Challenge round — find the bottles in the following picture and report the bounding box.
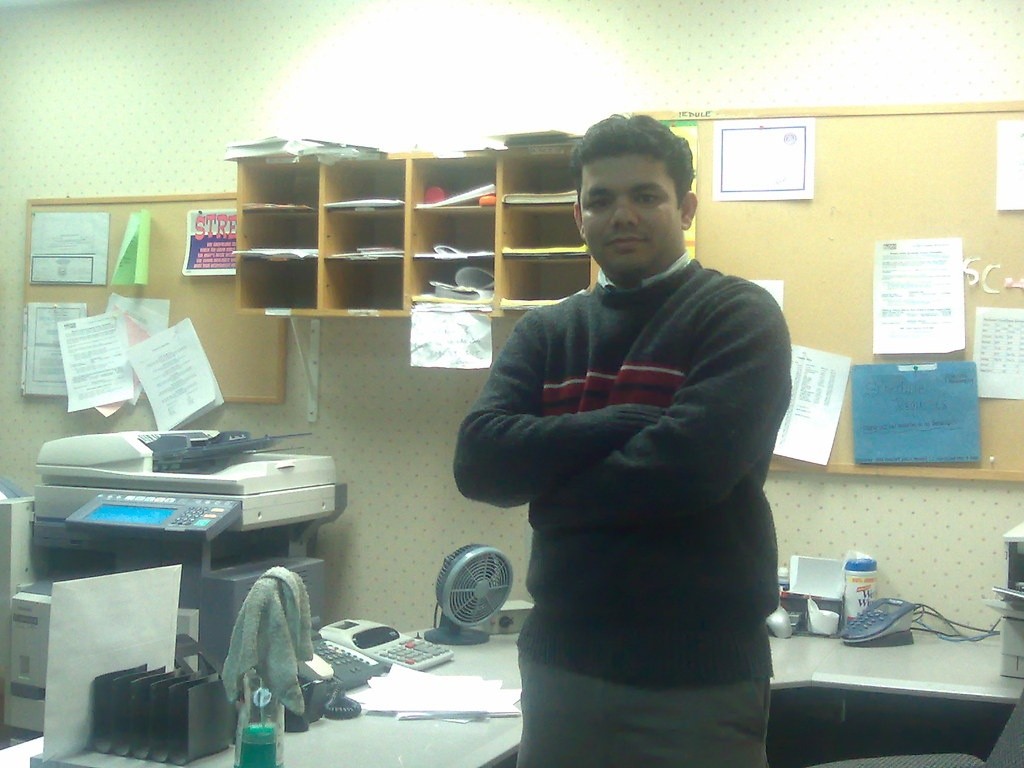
[777,568,789,591]
[235,673,285,768]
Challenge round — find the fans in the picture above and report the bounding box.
[424,545,513,645]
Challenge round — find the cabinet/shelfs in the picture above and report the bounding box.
[234,152,603,318]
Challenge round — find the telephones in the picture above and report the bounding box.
[297,629,373,694]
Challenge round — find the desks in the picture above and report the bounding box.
[0,625,1024,768]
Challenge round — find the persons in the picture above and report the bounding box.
[453,112,792,768]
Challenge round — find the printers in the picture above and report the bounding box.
[8,429,348,747]
[992,522,1024,679]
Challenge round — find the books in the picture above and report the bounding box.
[226,130,587,313]
